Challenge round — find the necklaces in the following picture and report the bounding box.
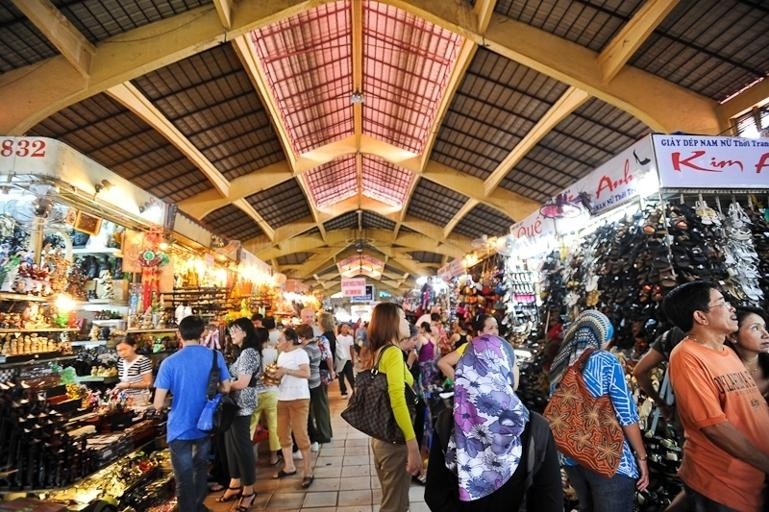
[739,354,762,372]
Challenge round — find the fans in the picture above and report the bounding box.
[471,232,495,254]
[493,232,522,256]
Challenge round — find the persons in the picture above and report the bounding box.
[659,279,769,512]
[722,305,768,404]
[631,324,690,512]
[108,337,152,403]
[154,302,647,512]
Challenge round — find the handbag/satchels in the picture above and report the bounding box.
[541,346,626,481]
[195,348,239,438]
[340,342,420,447]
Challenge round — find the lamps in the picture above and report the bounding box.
[92,178,113,201]
[209,235,228,249]
[137,201,158,218]
[538,187,595,220]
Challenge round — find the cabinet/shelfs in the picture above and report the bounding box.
[0,293,81,371]
[65,245,128,348]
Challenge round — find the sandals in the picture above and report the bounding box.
[215,485,242,503]
[235,490,258,512]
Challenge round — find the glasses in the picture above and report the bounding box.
[708,300,732,310]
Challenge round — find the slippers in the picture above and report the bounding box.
[268,454,315,488]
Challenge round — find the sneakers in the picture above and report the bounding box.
[292,450,303,460]
[310,442,319,452]
